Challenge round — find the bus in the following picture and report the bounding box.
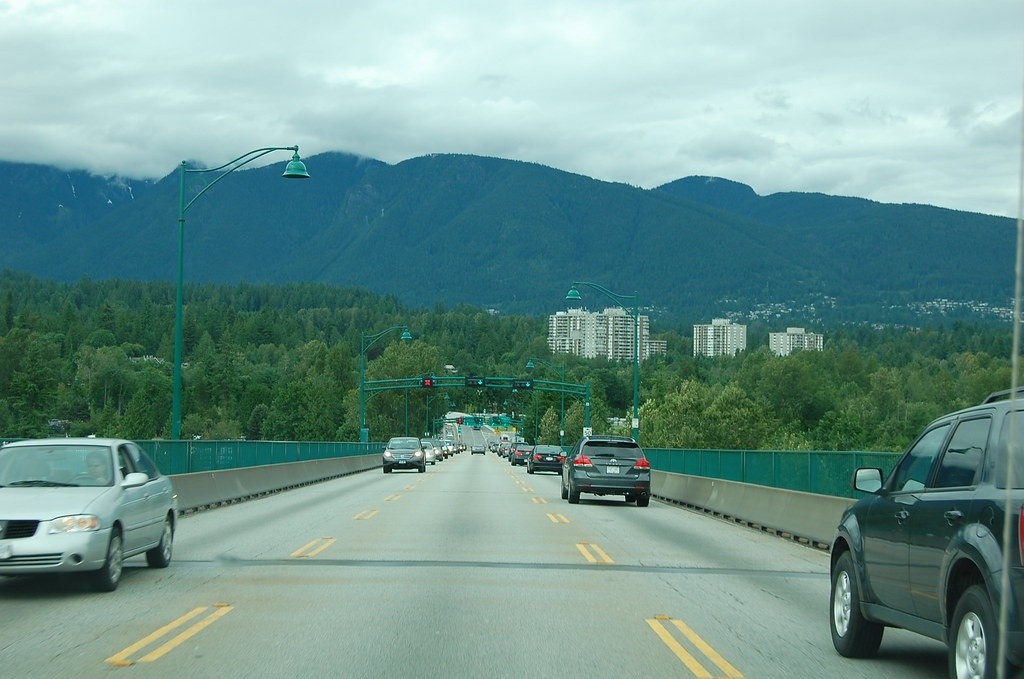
[560,435,652,507]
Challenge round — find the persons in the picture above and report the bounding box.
[72,451,113,486]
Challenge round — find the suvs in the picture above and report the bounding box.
[382,437,427,474]
[509,442,529,462]
[828,385,1024,679]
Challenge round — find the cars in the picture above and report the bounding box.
[488,441,509,456]
[526,444,565,474]
[439,440,449,459]
[426,437,443,461]
[504,446,511,458]
[511,445,535,467]
[0,437,179,591]
[471,445,486,455]
[420,441,437,465]
[443,425,467,455]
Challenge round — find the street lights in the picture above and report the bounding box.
[359,326,413,442]
[172,144,311,443]
[566,281,640,442]
[526,356,564,446]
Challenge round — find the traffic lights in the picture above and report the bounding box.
[458,418,462,424]
[513,378,534,388]
[422,377,434,387]
[465,376,485,387]
